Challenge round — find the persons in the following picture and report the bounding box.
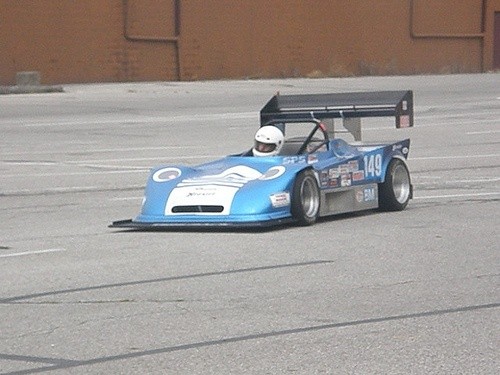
[252,124,285,158]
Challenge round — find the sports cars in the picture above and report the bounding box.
[107,90,415,231]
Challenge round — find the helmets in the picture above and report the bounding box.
[251,125,284,157]
